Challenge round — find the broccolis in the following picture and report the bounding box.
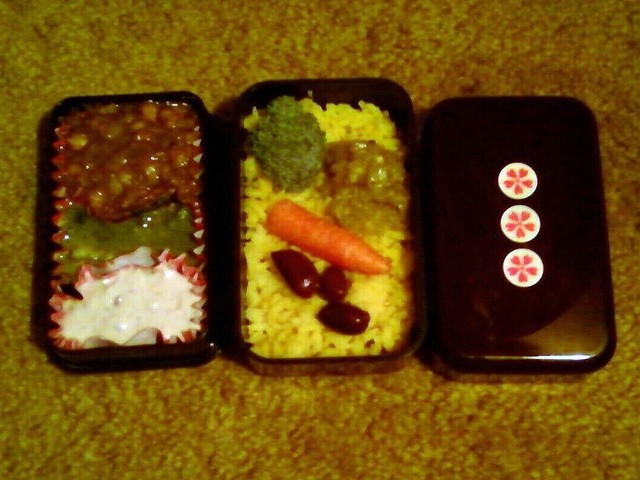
[254,97,325,193]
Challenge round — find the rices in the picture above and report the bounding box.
[239,95,414,359]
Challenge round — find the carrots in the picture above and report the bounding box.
[264,200,392,276]
